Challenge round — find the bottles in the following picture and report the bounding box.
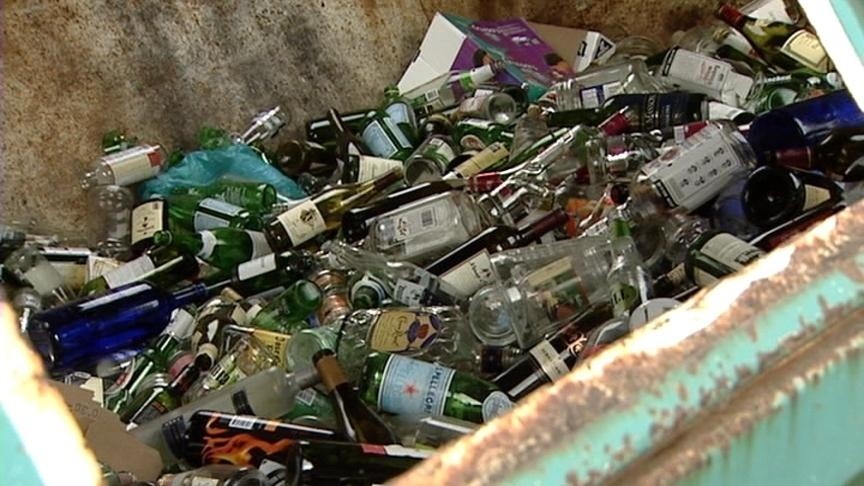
[2,1,863,485]
